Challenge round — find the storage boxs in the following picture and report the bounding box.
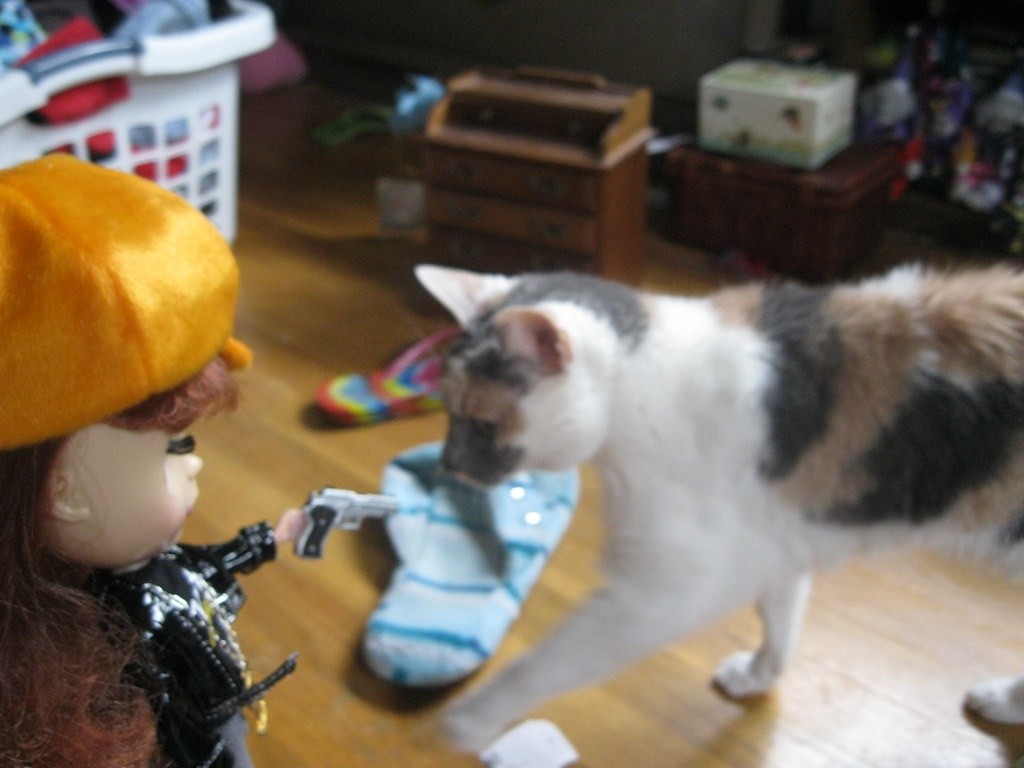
[668,136,904,289]
[700,59,859,162]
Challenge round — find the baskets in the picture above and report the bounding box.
[0,0,278,245]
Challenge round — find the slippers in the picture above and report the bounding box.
[361,441,576,686]
[315,326,471,426]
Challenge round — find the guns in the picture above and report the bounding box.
[293,485,401,559]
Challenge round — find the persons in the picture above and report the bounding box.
[0,151,304,768]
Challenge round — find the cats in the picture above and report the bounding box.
[406,259,1024,729]
